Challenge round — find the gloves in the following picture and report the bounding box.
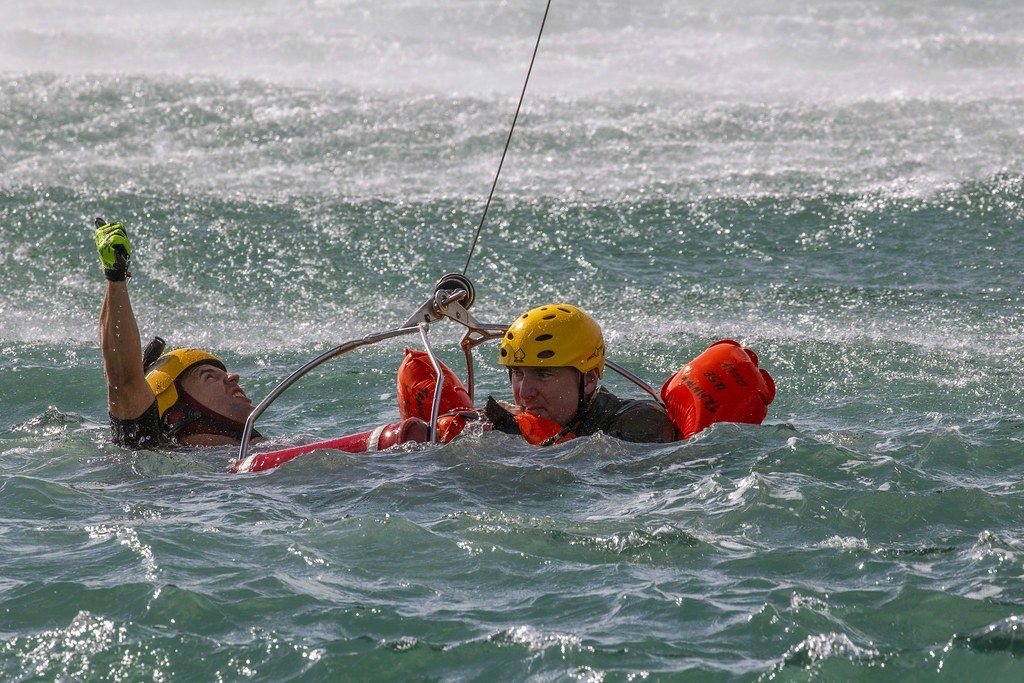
[95,217,131,281]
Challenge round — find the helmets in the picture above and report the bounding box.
[497,303,605,380]
[143,336,227,420]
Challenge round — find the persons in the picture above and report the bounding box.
[488,302,674,448]
[93,215,273,450]
